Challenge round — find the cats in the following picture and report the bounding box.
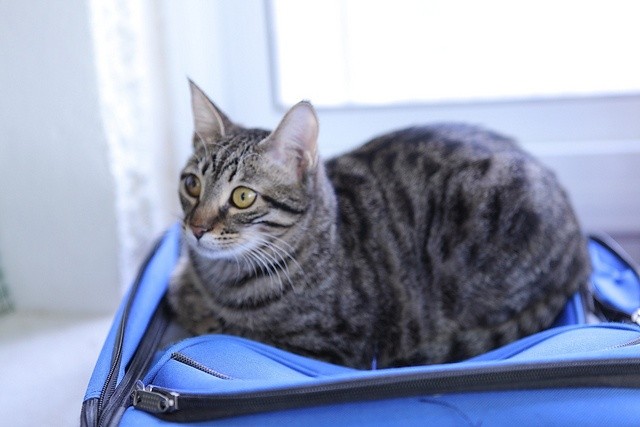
[166,76,594,372]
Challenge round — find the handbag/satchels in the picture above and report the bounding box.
[80,224,640,427]
